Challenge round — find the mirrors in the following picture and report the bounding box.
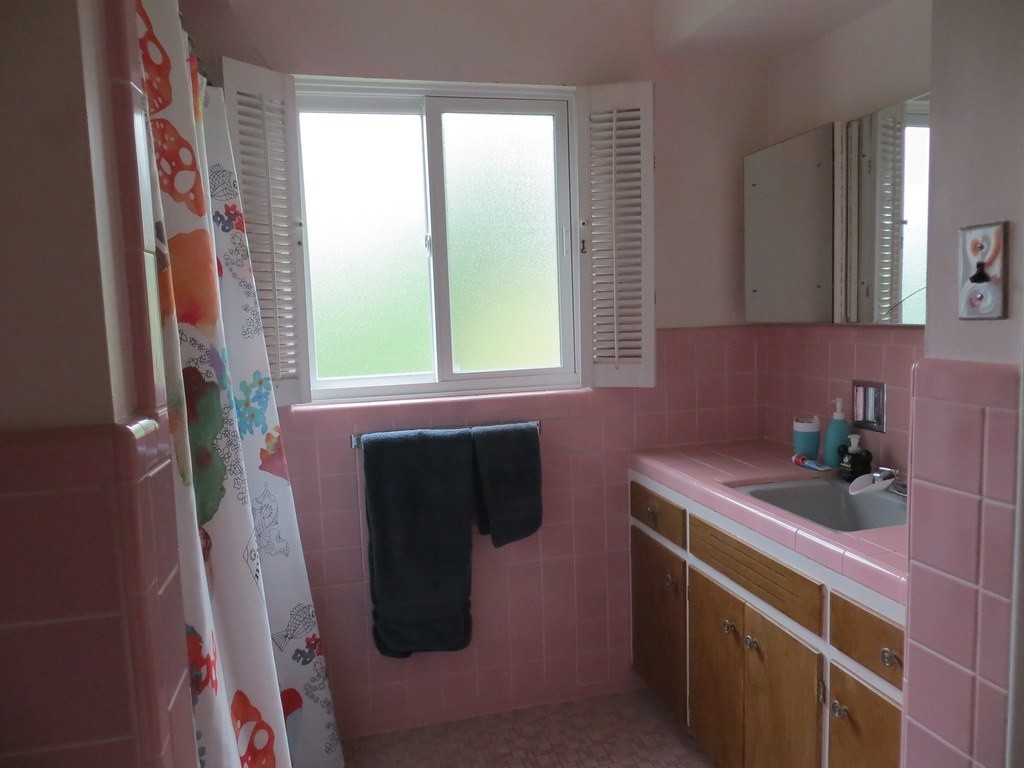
[845,91,931,326]
[743,122,835,324]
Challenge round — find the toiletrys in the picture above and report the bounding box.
[791,395,873,483]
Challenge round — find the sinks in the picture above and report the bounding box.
[730,475,908,534]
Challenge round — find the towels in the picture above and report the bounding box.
[360,421,544,660]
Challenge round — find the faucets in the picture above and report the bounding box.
[872,468,907,496]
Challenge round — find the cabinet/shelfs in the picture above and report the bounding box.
[686,510,826,768]
[627,468,687,729]
[826,569,907,768]
